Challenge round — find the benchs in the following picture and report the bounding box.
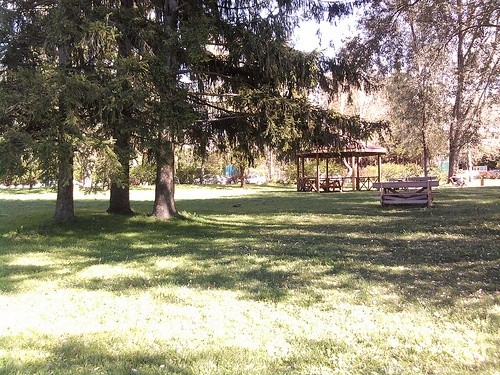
[318,179,343,193]
[372,181,440,211]
[388,175,438,203]
